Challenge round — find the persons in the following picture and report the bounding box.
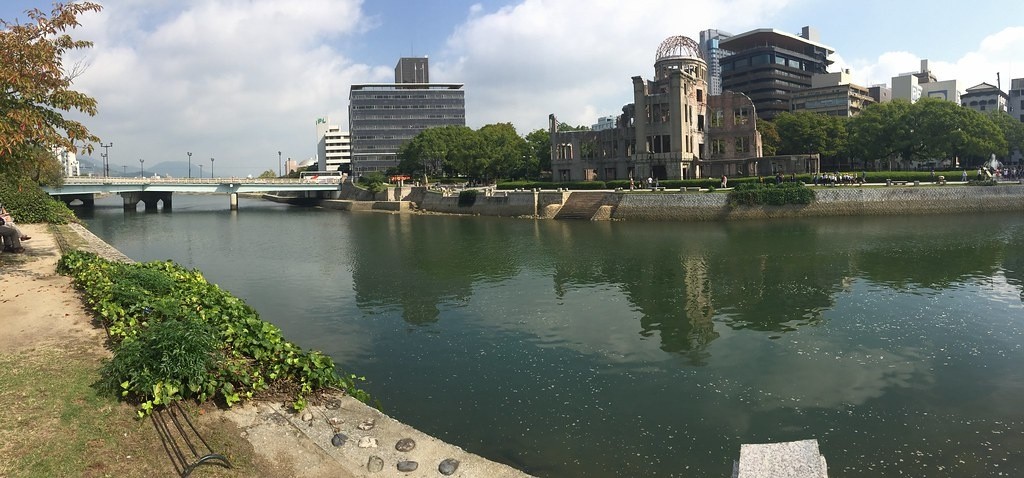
[630,176,659,190]
[721,175,727,188]
[0,207,32,254]
[435,179,476,187]
[759,171,866,187]
[931,164,1024,183]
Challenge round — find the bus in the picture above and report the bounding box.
[300,171,343,183]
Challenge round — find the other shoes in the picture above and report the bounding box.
[20,235,31,241]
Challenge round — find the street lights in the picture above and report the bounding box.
[100,142,113,177]
[187,152,193,178]
[140,158,145,178]
[211,158,214,178]
[278,150,282,177]
[101,152,107,177]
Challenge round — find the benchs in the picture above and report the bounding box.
[892,181,908,185]
[493,191,506,196]
[687,187,701,191]
[648,187,665,191]
